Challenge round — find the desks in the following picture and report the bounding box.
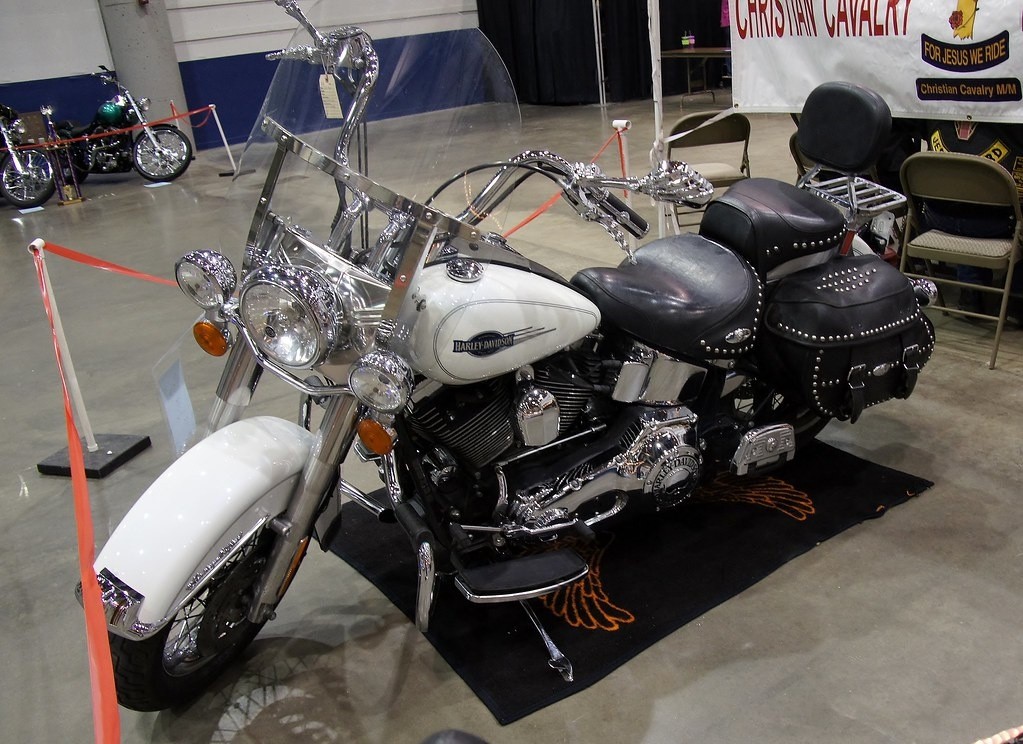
[661,48,732,110]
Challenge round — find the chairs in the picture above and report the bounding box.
[789,129,881,188]
[661,112,752,230]
[895,151,1023,370]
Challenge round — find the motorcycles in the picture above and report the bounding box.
[74,0,935,717]
[48,65,194,186]
[0,105,57,210]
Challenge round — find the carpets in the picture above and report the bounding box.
[326,435,937,726]
